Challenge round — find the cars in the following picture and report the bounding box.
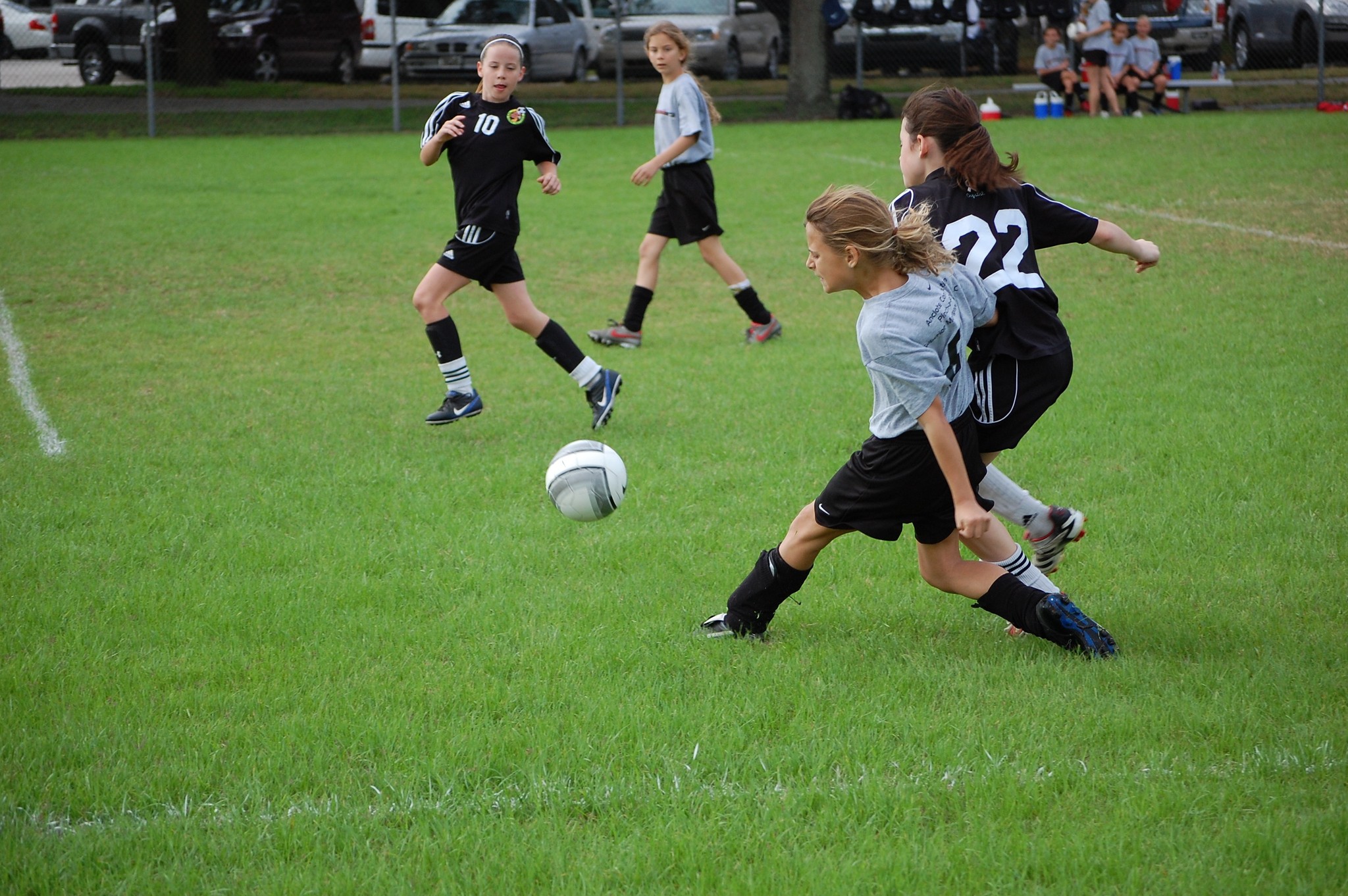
[1221,1,1348,69]
[401,0,593,84]
[565,0,627,66]
[1023,1,1230,70]
[50,0,170,85]
[138,0,365,88]
[834,0,1021,77]
[594,0,784,83]
[357,0,457,76]
[0,0,54,60]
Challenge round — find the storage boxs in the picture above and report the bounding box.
[1168,55,1182,79]
[1034,90,1065,118]
[981,97,1001,120]
[1164,90,1181,112]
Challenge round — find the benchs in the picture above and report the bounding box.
[1012,78,1233,112]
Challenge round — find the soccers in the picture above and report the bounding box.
[543,439,628,523]
[516,105,527,117]
[1064,20,1089,42]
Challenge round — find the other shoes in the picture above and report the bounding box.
[1131,109,1143,117]
[1081,100,1090,111]
[1101,110,1109,117]
[1064,109,1074,115]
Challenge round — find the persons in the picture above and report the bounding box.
[413,36,622,429]
[588,19,785,350]
[699,185,1121,663]
[888,87,1160,637]
[1034,0,1167,116]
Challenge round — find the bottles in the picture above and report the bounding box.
[1218,61,1227,80]
[1211,61,1217,79]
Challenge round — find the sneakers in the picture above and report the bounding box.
[746,312,782,341]
[1002,621,1025,637]
[1024,505,1089,577]
[700,613,763,639]
[588,318,641,348]
[424,388,482,425]
[1036,593,1120,658]
[584,368,623,429]
